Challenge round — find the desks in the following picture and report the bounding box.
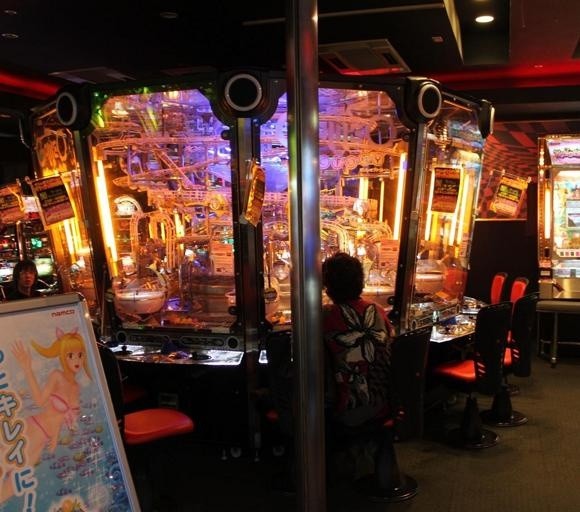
[536,300,580,367]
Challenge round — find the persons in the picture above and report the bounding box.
[322,252,395,491]
[0,262,45,302]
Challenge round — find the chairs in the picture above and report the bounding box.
[100,346,197,512]
